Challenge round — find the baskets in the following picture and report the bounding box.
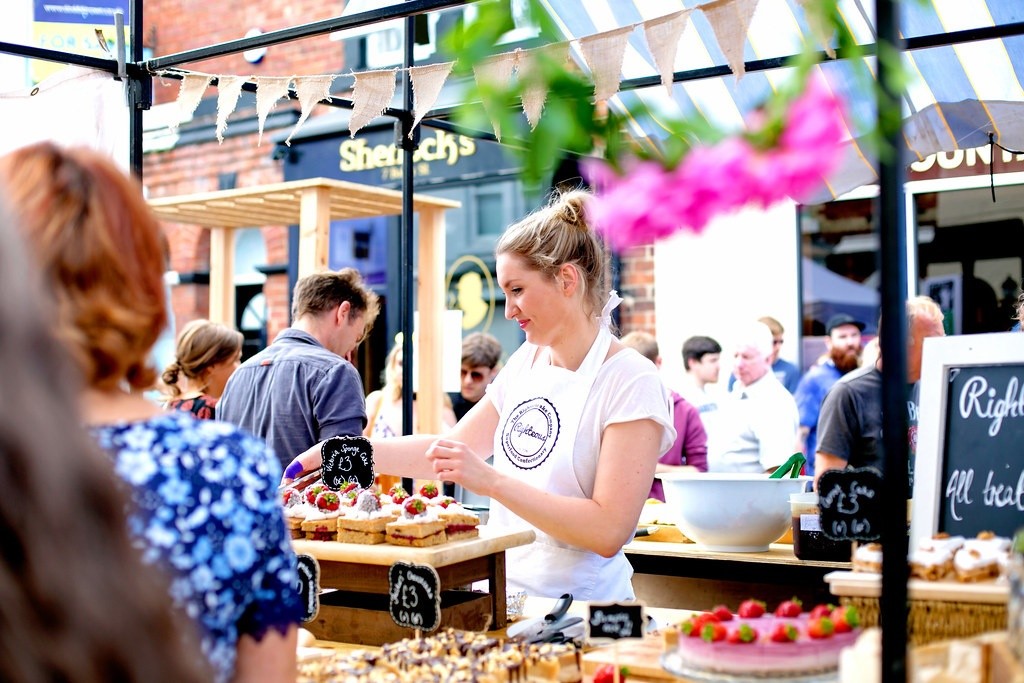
[836,594,1008,655]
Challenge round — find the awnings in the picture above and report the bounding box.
[541,0,1024,205]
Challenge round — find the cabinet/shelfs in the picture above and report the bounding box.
[291,527,535,649]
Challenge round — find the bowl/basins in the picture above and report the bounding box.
[655,472,808,552]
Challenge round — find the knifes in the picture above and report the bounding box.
[506,593,573,643]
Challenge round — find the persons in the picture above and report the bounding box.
[281,188,676,601]
[0,138,304,683]
[816,299,946,496]
[362,333,502,437]
[216,270,379,472]
[162,320,243,420]
[0,191,210,683]
[621,315,869,505]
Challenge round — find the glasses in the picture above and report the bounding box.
[461,368,491,383]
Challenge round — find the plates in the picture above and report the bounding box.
[507,616,586,644]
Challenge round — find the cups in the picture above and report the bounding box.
[789,493,851,561]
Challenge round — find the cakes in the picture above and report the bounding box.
[666,600,865,683]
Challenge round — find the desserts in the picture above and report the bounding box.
[852,542,882,574]
[280,483,480,548]
[908,532,1012,583]
[296,627,582,683]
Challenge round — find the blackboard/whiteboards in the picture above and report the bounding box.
[909,331,1024,554]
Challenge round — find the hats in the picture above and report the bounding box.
[825,313,866,336]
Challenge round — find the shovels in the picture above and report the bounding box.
[510,592,573,643]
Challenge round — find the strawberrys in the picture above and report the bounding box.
[593,665,626,683]
